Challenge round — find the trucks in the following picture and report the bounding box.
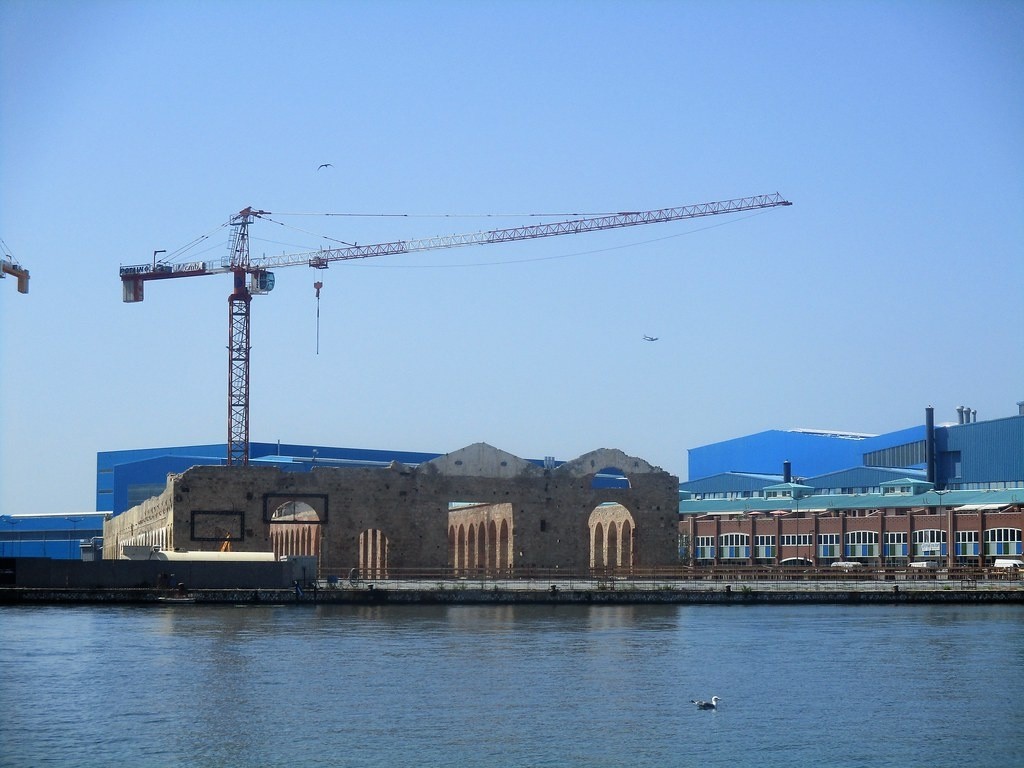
[906,562,940,568]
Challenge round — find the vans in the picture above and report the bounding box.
[994,559,1024,567]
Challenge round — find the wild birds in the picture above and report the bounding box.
[690,696,722,710]
[317,163,336,171]
[642,333,661,342]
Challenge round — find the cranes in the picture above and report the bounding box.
[118,189,793,469]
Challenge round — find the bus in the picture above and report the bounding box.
[831,561,862,568]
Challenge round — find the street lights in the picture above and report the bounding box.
[786,492,811,580]
[929,488,952,578]
